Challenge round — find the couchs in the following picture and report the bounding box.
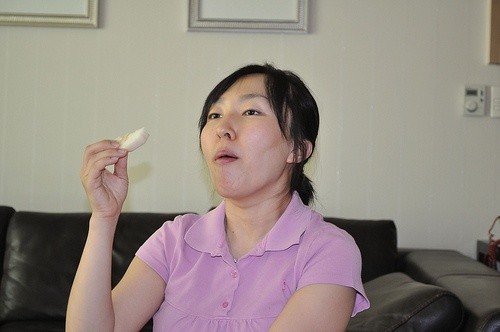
[0,206,500,332]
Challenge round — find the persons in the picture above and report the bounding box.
[64,63,371,332]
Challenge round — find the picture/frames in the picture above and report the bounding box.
[0,0,98,30]
[183,0,310,35]
